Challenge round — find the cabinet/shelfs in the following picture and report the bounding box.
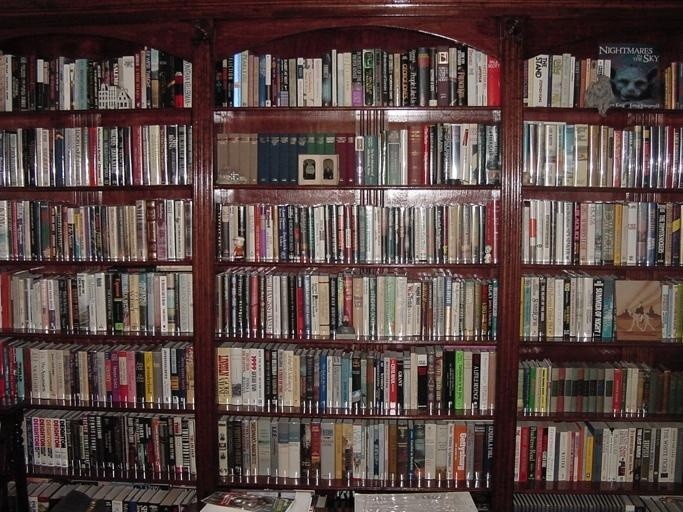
[0,1,202,496]
[513,0,683,496]
[200,2,511,497]
